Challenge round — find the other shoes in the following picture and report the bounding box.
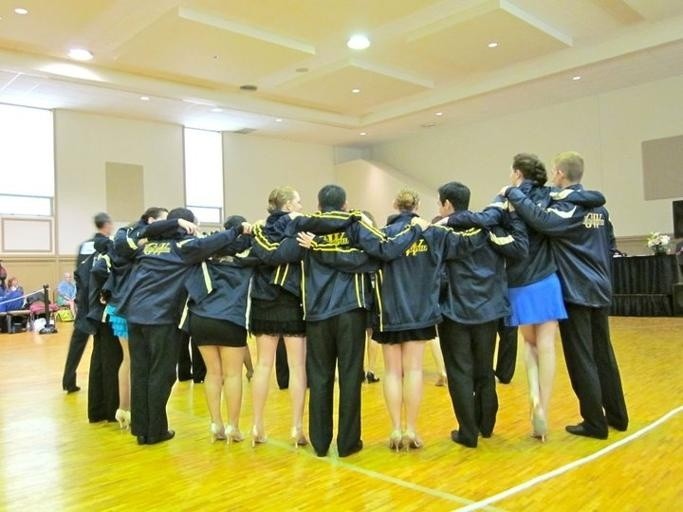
[89,415,116,423]
[314,447,329,456]
[340,440,362,456]
[138,430,175,444]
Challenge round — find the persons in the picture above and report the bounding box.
[250,152,630,458]
[62,187,362,450]
[1,266,24,312]
[57,272,77,319]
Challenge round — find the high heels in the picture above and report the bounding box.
[250,425,266,447]
[434,372,447,387]
[367,371,380,383]
[291,429,307,448]
[209,422,227,443]
[389,430,403,452]
[402,431,420,453]
[115,410,131,430]
[224,424,245,445]
[532,417,548,442]
[245,371,252,383]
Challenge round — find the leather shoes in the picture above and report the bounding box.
[63,383,80,392]
[604,416,627,431]
[566,422,607,440]
[452,430,477,448]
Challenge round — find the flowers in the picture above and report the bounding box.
[639,231,672,248]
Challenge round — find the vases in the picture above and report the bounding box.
[653,246,669,255]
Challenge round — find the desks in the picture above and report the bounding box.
[608,253,682,318]
